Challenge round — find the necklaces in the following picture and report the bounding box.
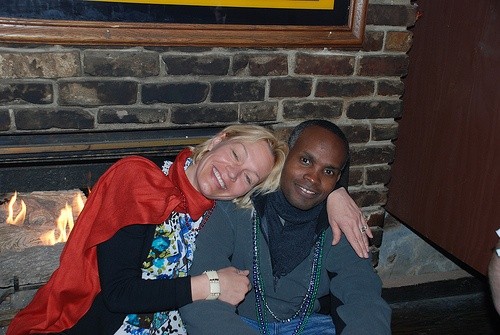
[173,187,217,232]
[252,209,326,335]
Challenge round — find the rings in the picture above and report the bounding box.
[360,225,367,233]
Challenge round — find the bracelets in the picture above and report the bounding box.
[202,270,221,301]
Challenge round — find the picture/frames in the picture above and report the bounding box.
[0,0,368,49]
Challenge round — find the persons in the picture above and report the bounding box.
[6,123,374,335]
[178,120,391,335]
